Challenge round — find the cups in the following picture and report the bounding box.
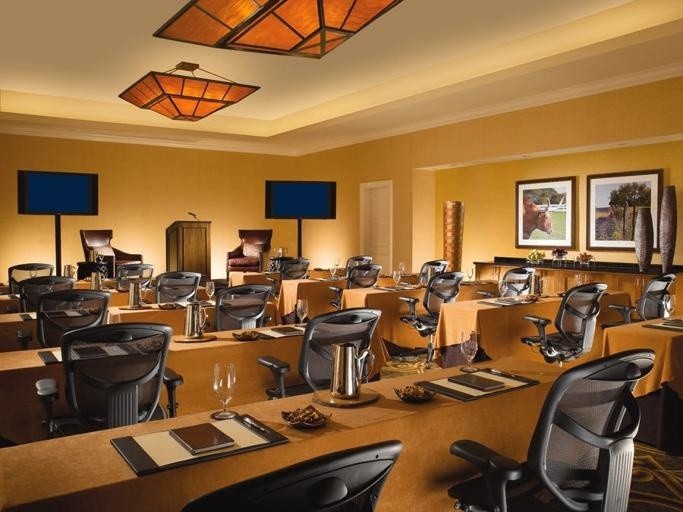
[278,247,289,257]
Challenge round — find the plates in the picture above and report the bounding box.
[232,331,265,341]
[279,404,333,429]
[117,289,129,293]
[158,304,176,309]
[394,383,437,403]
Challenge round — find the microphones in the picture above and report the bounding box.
[188,212,201,222]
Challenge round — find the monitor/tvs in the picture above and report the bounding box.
[16,168,99,216]
[263,178,337,219]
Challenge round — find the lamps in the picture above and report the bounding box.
[117,70,261,123]
[150,1,400,61]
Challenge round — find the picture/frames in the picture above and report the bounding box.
[514,175,575,249]
[586,168,663,252]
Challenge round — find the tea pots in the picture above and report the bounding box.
[63,264,76,277]
[183,302,209,339]
[330,341,375,399]
[127,280,147,308]
[527,273,547,299]
[89,271,106,291]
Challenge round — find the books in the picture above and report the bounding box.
[271,326,303,336]
[46,311,68,318]
[448,373,504,392]
[169,423,235,455]
[73,345,107,359]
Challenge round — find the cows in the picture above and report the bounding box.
[523,196,553,239]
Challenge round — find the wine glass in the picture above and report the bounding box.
[106,311,121,325]
[330,255,341,278]
[296,299,309,326]
[460,330,478,372]
[393,263,405,287]
[662,294,678,322]
[211,362,237,419]
[466,267,474,282]
[498,280,508,300]
[206,281,215,302]
[30,267,38,279]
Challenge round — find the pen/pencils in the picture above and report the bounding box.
[490,370,516,379]
[243,417,270,433]
